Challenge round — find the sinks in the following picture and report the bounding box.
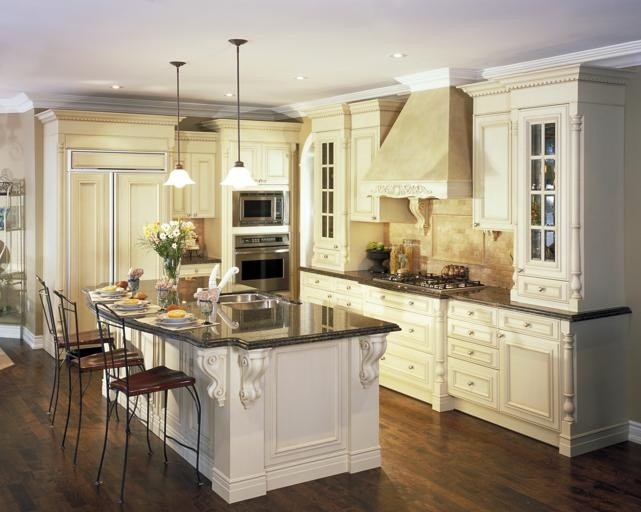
[217,291,269,304]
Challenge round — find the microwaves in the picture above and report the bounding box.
[232,190,290,226]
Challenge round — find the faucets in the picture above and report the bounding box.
[208,262,240,302]
[209,303,240,336]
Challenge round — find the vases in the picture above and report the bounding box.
[158,255,186,314]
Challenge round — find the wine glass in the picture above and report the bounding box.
[199,300,214,325]
[157,289,169,313]
[366,249,390,273]
[127,278,140,299]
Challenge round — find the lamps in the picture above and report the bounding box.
[163,60,197,188]
[219,36,258,190]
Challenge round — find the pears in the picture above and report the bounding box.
[135,291,147,300]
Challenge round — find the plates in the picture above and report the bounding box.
[114,300,144,307]
[97,288,124,295]
[94,292,125,297]
[161,313,191,322]
[109,304,150,310]
[153,319,198,325]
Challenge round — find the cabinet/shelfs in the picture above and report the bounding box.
[493,307,631,459]
[361,276,488,418]
[171,128,216,220]
[296,264,394,319]
[346,98,416,224]
[457,72,517,237]
[195,119,304,194]
[443,296,496,419]
[38,113,176,364]
[308,104,385,274]
[507,64,630,318]
[0,179,26,346]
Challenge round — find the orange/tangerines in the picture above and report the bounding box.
[366,242,390,252]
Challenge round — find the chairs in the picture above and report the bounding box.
[51,287,152,465]
[92,300,208,509]
[30,271,120,428]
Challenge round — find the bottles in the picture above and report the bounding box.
[389,238,418,272]
[443,263,470,278]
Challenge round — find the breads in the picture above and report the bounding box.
[167,309,186,319]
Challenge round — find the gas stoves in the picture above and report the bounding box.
[373,269,486,294]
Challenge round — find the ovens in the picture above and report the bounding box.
[232,234,290,292]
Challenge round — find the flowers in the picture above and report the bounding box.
[134,216,205,285]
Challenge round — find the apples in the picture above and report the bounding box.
[119,281,128,288]
